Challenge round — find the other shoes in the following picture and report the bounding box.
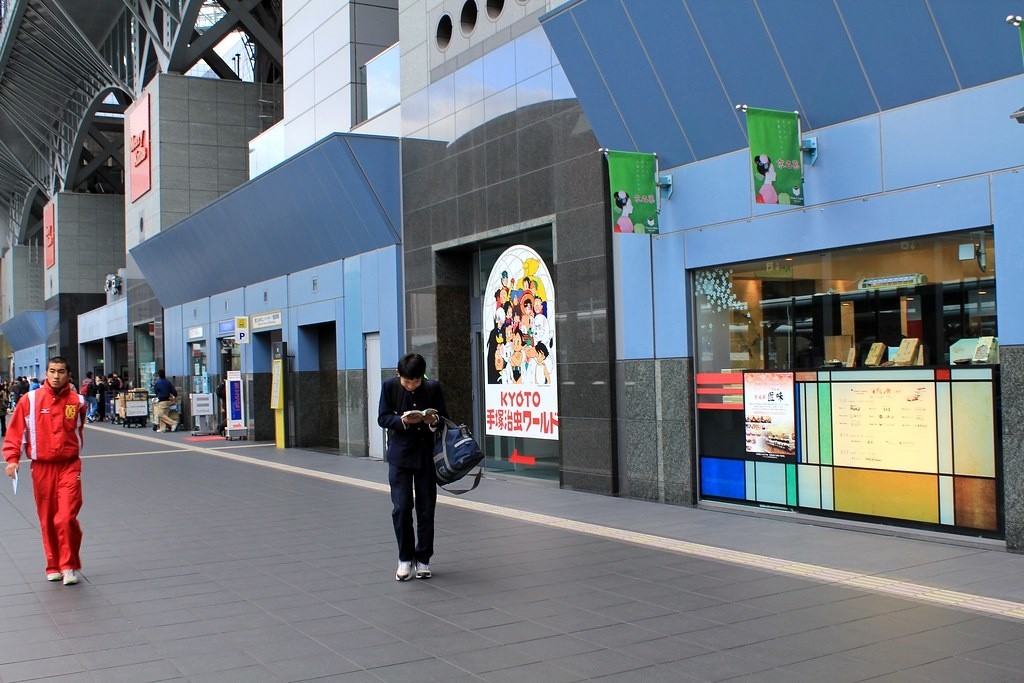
[96,418,103,421]
[156,429,166,433]
[87,415,94,423]
[171,421,178,432]
[110,418,120,424]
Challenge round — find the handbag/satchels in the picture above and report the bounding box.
[431,423,486,487]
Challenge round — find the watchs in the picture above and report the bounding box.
[431,414,438,426]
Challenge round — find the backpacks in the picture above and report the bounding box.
[78,381,91,398]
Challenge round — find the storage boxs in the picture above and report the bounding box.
[894,338,921,365]
[846,348,856,367]
[972,336,997,363]
[865,342,886,367]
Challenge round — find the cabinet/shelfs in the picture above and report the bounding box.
[113,389,148,427]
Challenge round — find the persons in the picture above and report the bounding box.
[1,356,87,586]
[67,371,124,424]
[377,353,446,582]
[0,376,45,437]
[153,369,179,433]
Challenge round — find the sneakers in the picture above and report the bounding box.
[395,557,416,581]
[62,569,79,585]
[47,572,61,581]
[414,561,431,578]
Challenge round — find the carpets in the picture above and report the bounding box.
[180,435,226,441]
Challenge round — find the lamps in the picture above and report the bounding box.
[858,273,927,290]
